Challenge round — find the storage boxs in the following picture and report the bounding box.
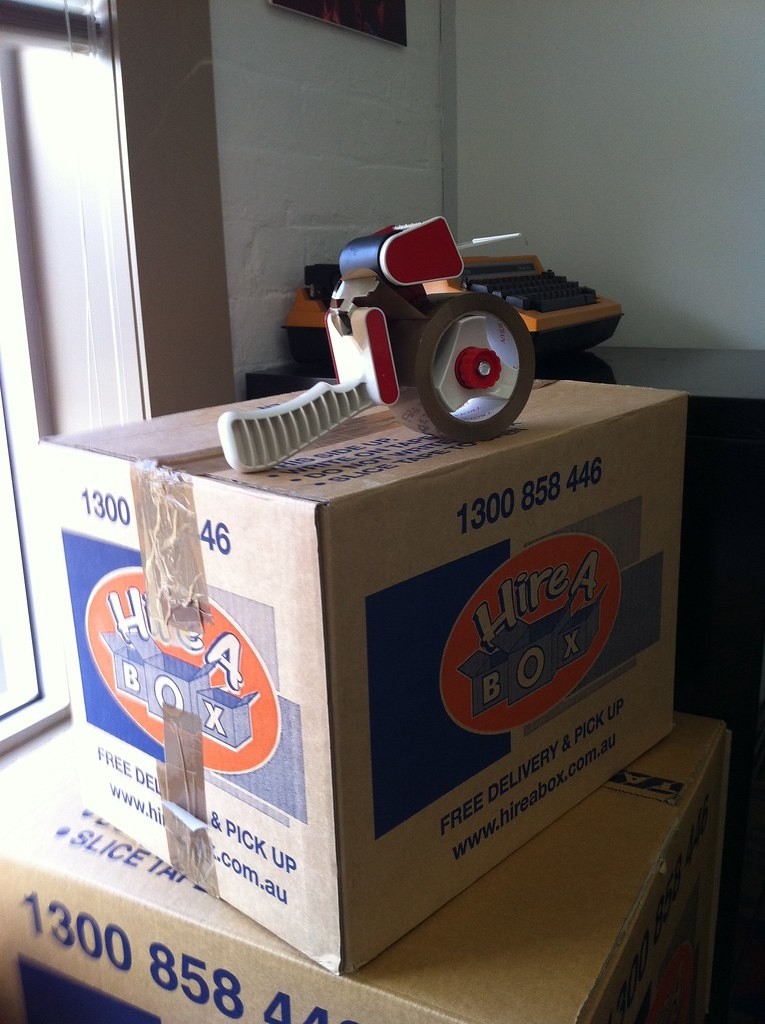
[2,682,738,1024]
[35,361,698,985]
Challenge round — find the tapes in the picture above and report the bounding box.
[387,292,535,444]
